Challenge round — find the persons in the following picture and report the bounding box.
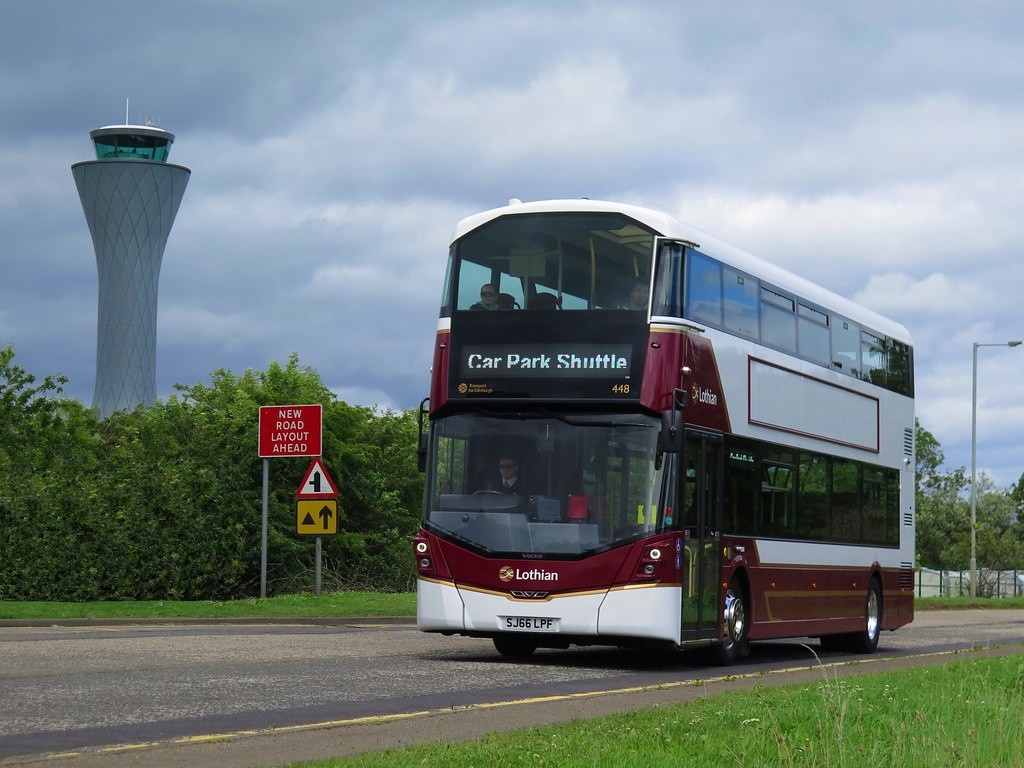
[616,276,650,310]
[483,450,529,514]
[469,284,507,311]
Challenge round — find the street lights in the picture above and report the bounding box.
[967,339,1022,599]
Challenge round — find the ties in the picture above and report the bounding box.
[504,482,509,488]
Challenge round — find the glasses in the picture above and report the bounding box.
[480,290,498,297]
[500,465,516,470]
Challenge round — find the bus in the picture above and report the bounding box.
[413,199,917,666]
